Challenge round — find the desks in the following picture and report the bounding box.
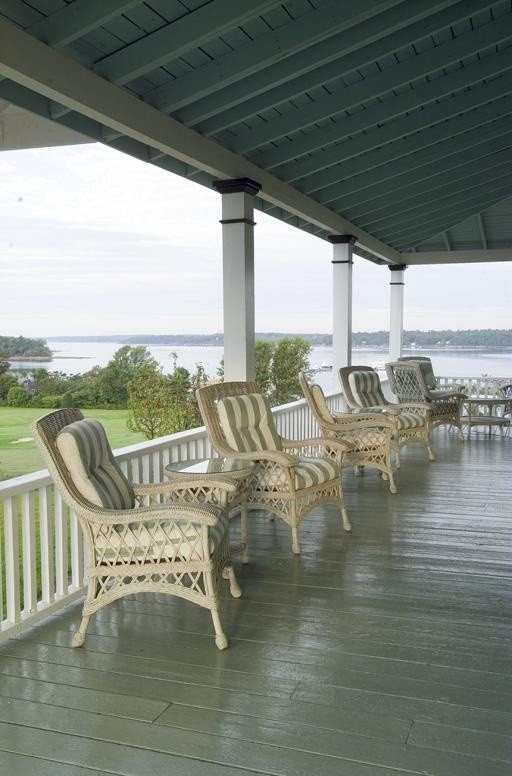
[161,454,255,564]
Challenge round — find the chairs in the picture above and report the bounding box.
[498,380,512,427]
[385,355,465,439]
[297,371,401,494]
[36,405,244,650]
[195,380,352,554]
[338,364,435,469]
